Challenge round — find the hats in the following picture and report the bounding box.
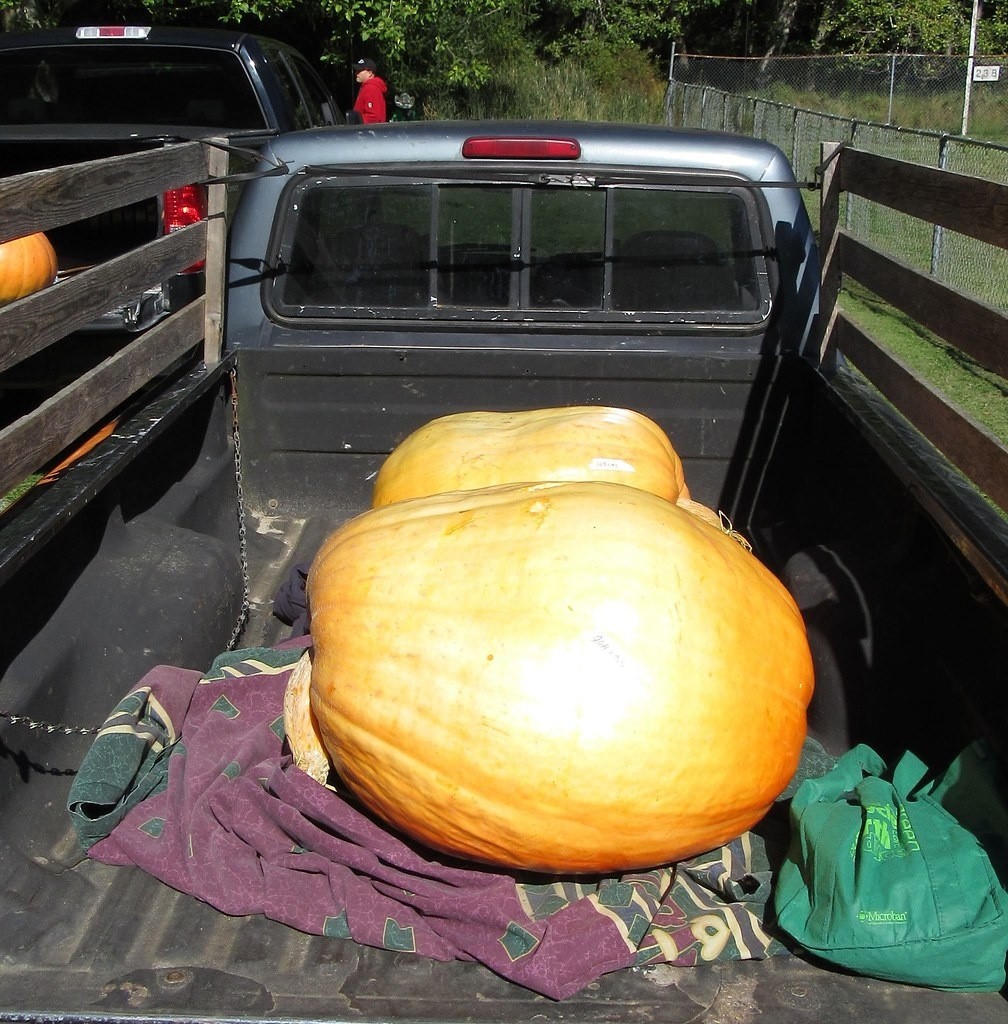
[352,59,377,72]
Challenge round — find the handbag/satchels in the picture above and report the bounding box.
[773,740,1008,992]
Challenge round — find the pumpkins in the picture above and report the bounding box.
[0,232,58,301]
[282,407,816,874]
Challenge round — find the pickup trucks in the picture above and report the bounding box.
[0,125,1008,1023]
[0,18,363,342]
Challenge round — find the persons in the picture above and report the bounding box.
[351,58,386,125]
